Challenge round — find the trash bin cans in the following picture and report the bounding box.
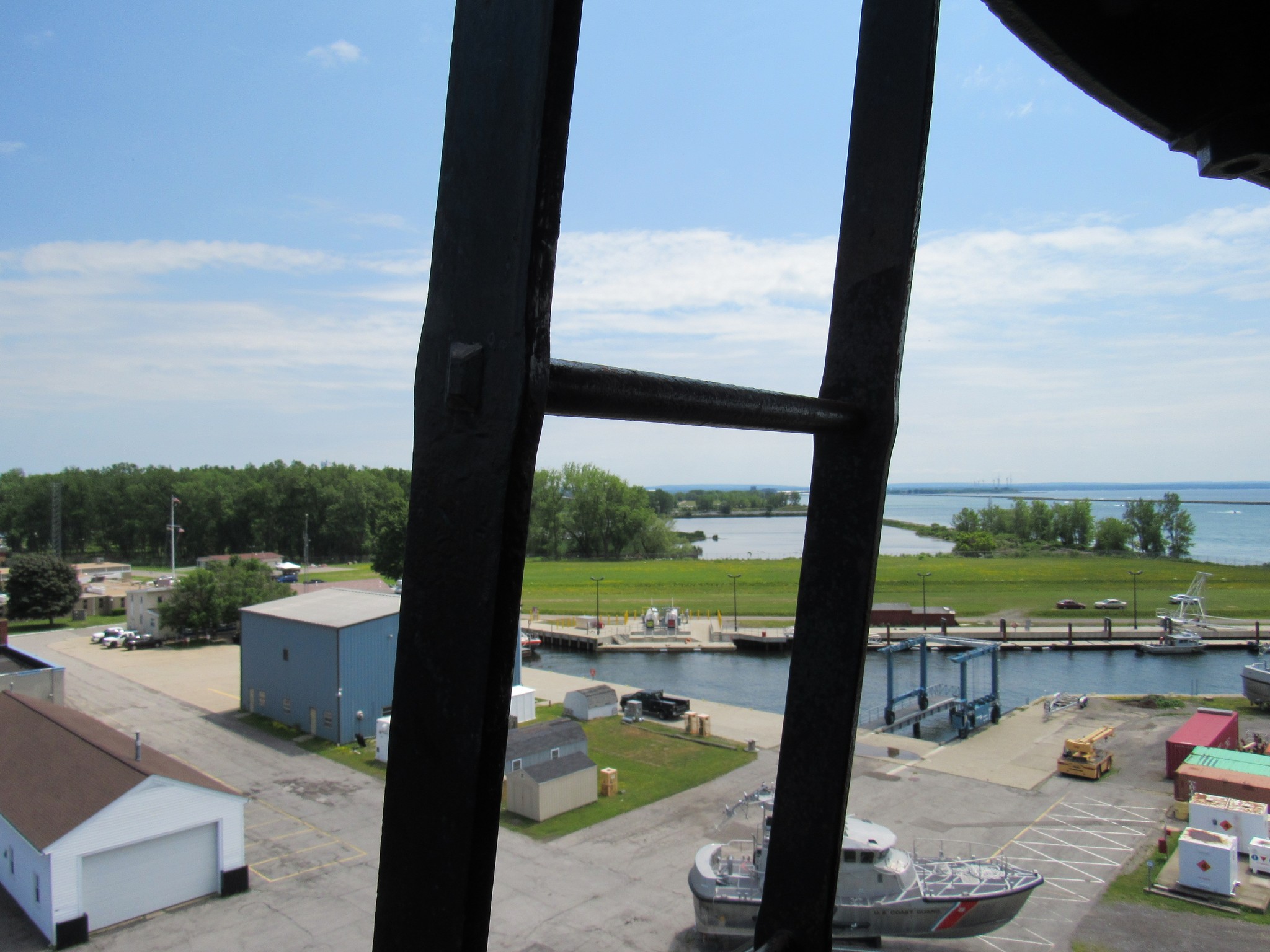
[762,631,766,636]
[1158,838,1167,853]
[1174,798,1189,820]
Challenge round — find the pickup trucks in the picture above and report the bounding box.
[620,689,690,721]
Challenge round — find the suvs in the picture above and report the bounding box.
[276,575,298,584]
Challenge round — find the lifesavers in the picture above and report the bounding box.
[685,639,691,644]
[589,668,596,676]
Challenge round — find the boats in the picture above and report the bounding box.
[1140,628,1208,653]
[687,782,1045,952]
[520,631,542,653]
[1240,659,1270,710]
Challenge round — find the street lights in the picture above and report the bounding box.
[915,572,932,631]
[165,493,185,578]
[727,574,743,631]
[1128,570,1143,629]
[590,576,605,635]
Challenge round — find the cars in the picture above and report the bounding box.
[91,627,163,651]
[1169,594,1200,605]
[1055,599,1086,609]
[303,579,327,584]
[1094,599,1127,610]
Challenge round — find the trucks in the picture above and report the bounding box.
[394,579,402,594]
